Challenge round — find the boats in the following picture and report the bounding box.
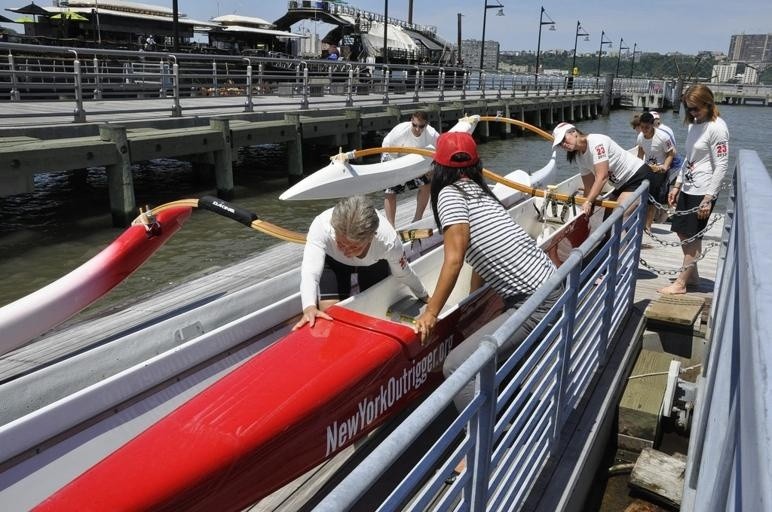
[15,145,645,511]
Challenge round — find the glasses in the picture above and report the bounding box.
[687,106,699,112]
[412,123,426,128]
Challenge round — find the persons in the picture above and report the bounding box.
[628,109,683,238]
[137,33,159,52]
[655,83,730,296]
[289,193,432,335]
[319,44,340,60]
[551,122,654,287]
[412,129,567,485]
[379,110,445,229]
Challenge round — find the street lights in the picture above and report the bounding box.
[617,37,630,79]
[478,1,505,87]
[596,31,612,92]
[534,6,556,89]
[567,20,590,91]
[631,42,642,78]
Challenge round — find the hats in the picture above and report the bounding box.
[433,131,479,166]
[650,111,660,119]
[552,121,573,147]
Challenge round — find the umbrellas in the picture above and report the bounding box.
[15,16,36,25]
[0,14,17,43]
[10,0,53,39]
[49,7,90,22]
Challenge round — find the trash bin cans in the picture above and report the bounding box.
[394,84,405,94]
[567,76,573,89]
[357,84,369,94]
[310,84,324,97]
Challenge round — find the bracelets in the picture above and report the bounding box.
[704,194,713,199]
[426,308,438,319]
[671,186,680,191]
[585,201,592,206]
